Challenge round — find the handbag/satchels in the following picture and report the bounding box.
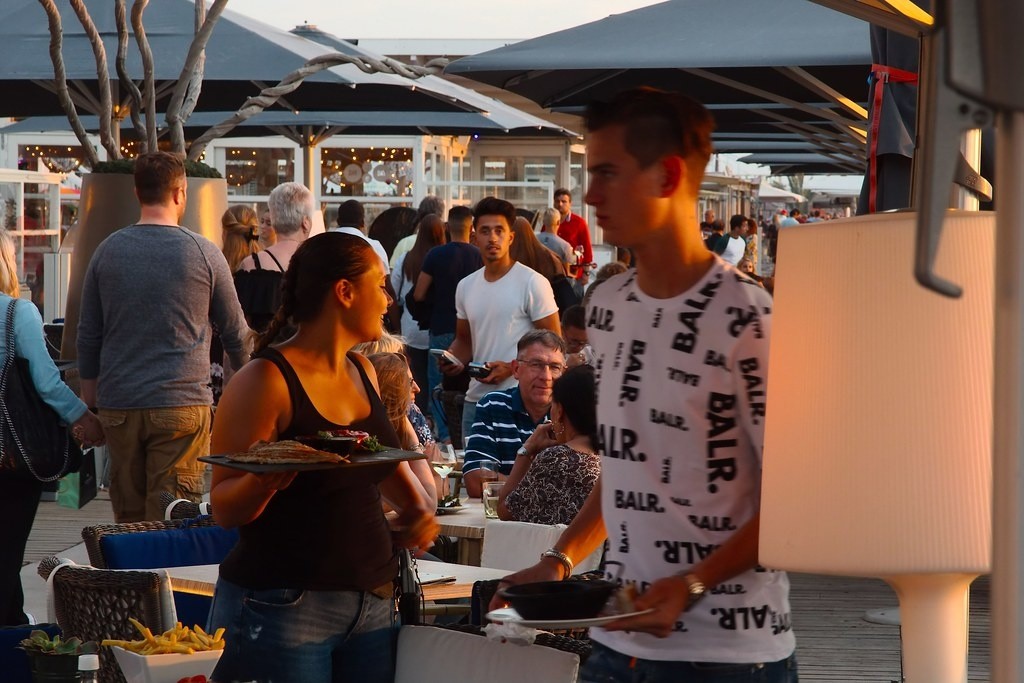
[384,301,403,334]
[1,298,85,486]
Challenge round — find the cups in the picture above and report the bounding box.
[482,480,508,519]
[479,461,498,502]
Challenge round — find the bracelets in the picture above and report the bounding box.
[407,443,427,453]
[540,550,574,582]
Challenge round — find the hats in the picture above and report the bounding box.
[337,200,365,225]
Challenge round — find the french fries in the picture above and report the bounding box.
[101,617,225,655]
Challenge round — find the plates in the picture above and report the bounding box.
[436,504,471,513]
[485,608,655,630]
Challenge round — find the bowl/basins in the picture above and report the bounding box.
[109,644,224,683]
[496,581,621,621]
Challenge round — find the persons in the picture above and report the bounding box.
[210,230,438,683]
[0,225,107,627]
[74,152,257,523]
[206,183,843,634]
[488,74,802,683]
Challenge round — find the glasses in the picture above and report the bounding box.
[518,359,561,374]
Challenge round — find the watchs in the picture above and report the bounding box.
[676,568,707,613]
[517,446,535,465]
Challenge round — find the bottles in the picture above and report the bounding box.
[77,654,99,683]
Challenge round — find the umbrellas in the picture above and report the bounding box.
[0,0,997,217]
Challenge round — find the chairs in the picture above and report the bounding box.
[35,489,242,683]
[394,620,593,683]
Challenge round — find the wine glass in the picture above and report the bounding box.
[430,442,456,502]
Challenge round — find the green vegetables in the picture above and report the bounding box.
[316,431,389,453]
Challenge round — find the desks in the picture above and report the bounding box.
[149,562,517,634]
[379,491,501,567]
[480,518,608,581]
